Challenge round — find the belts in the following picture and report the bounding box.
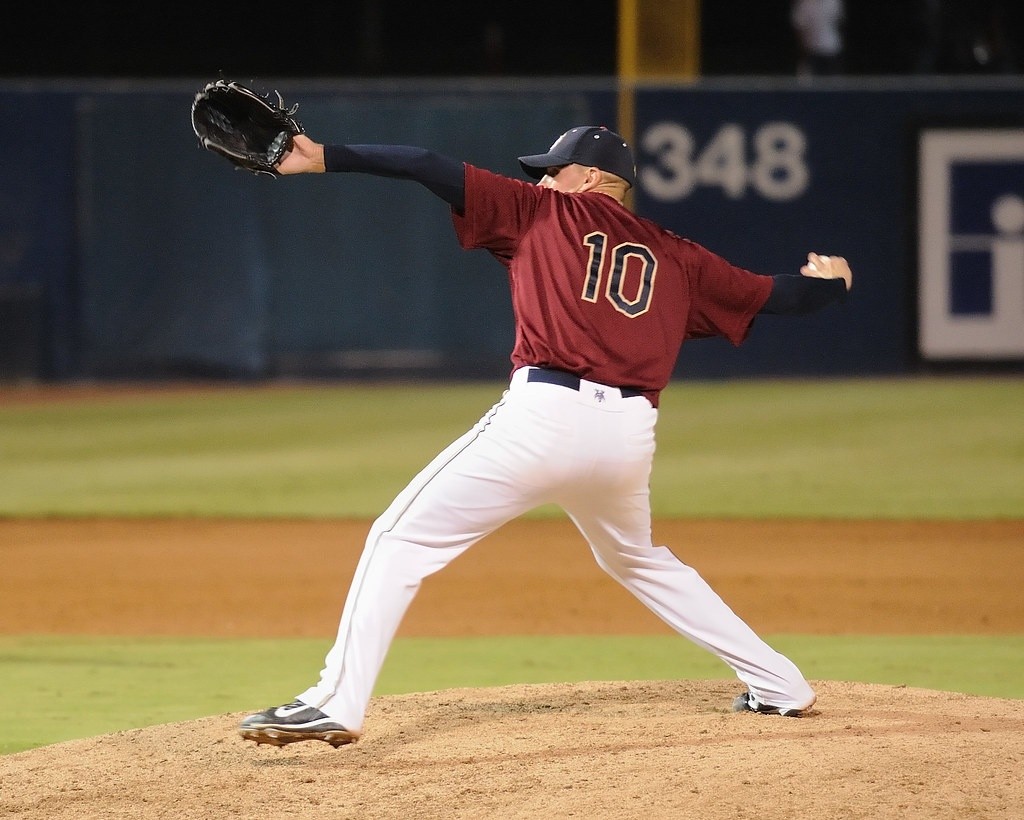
[528,368,642,398]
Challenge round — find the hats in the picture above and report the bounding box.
[518,124,634,190]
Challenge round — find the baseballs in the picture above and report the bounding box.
[807,255,830,271]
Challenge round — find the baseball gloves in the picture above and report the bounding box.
[191,79,306,176]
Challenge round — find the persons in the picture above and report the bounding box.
[238,126,852,749]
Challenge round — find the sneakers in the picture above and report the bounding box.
[240,699,357,750]
[733,690,816,718]
